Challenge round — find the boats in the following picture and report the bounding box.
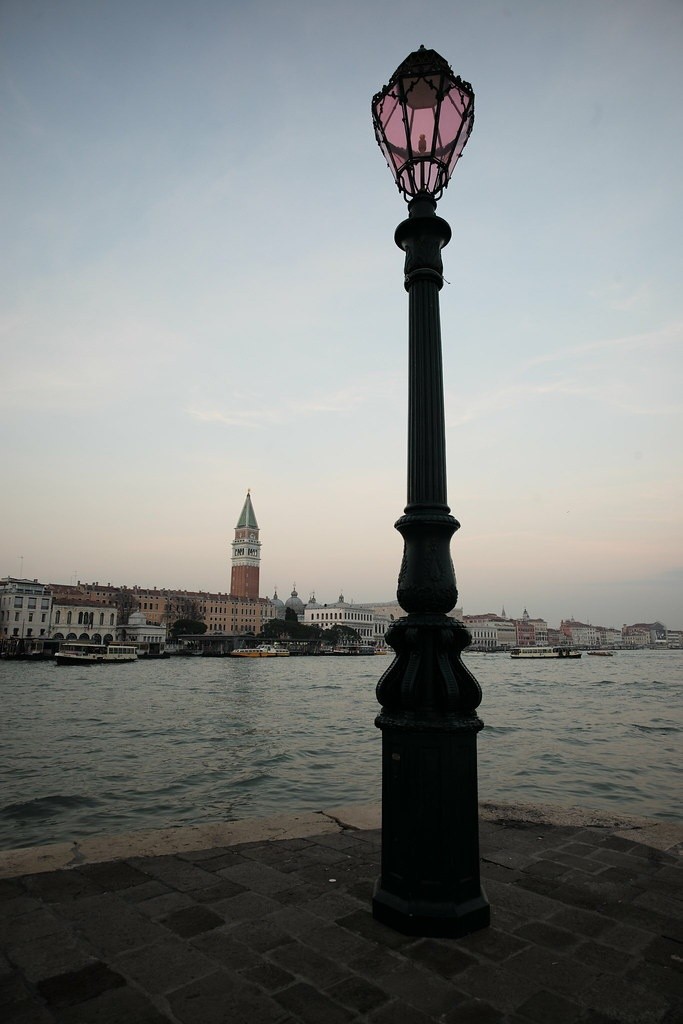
[230,645,289,658]
[54,643,138,666]
[587,652,612,656]
[510,646,583,659]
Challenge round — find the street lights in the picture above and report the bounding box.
[371,44,486,941]
